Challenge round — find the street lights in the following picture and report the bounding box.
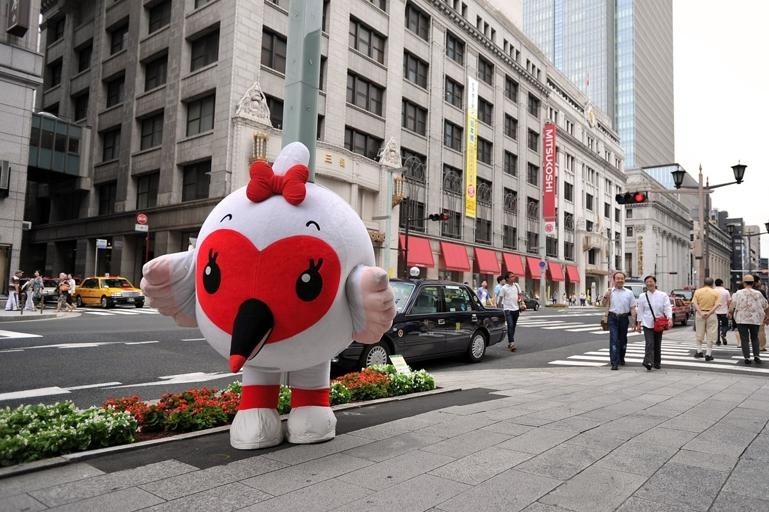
[727,222,769,274]
[671,161,748,289]
[409,267,420,279]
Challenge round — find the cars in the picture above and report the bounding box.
[521,292,540,312]
[19,276,80,299]
[668,296,691,326]
[75,275,145,308]
[329,279,506,371]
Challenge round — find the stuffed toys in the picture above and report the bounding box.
[139,141,397,451]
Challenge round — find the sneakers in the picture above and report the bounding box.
[611,365,617,370]
[510,342,517,352]
[694,350,703,357]
[647,364,651,370]
[705,355,713,361]
[654,364,661,369]
[754,357,761,362]
[744,358,752,364]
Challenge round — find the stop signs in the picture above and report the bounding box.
[137,213,148,224]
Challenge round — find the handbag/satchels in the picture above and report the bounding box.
[518,293,526,313]
[654,316,668,332]
[600,316,609,331]
[60,283,71,291]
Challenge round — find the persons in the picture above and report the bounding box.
[636,275,674,370]
[730,275,769,364]
[731,315,742,348]
[3,269,78,313]
[497,271,524,352]
[753,276,769,351]
[602,271,637,371]
[692,277,720,361]
[714,279,731,345]
[493,275,506,308]
[552,288,590,308]
[478,279,491,307]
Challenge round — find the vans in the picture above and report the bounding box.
[623,282,659,315]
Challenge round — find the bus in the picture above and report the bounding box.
[670,289,694,313]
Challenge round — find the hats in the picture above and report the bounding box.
[743,274,754,282]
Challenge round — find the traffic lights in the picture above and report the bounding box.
[429,213,449,221]
[615,191,647,204]
